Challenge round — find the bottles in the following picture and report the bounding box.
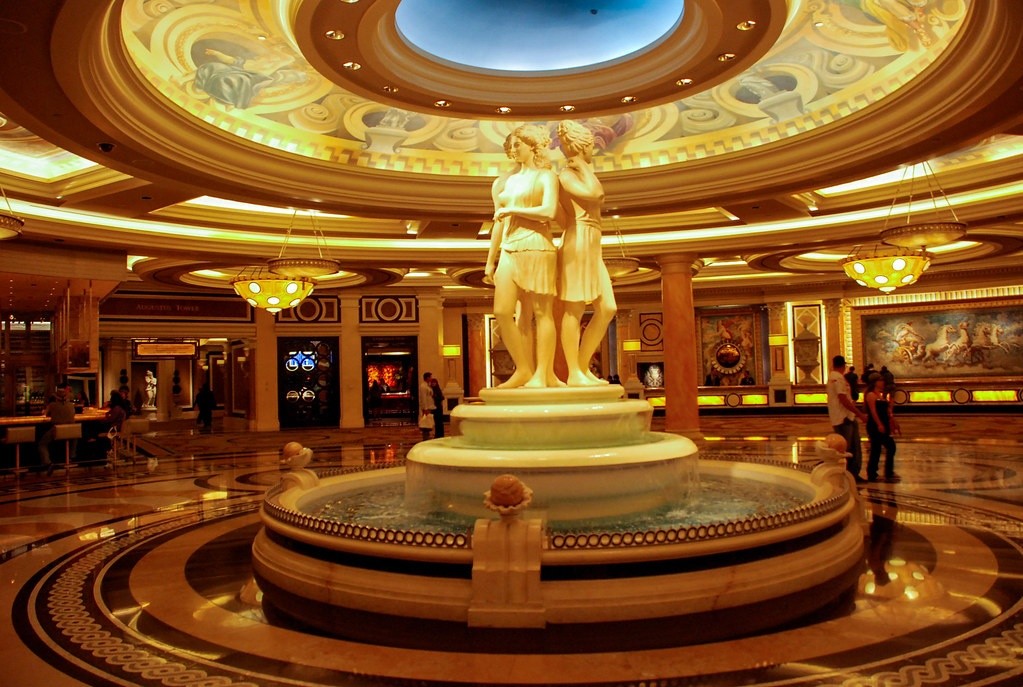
[24,386,45,402]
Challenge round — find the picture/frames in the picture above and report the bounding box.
[850,294,1023,384]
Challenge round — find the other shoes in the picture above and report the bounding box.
[867,473,880,481]
[48,462,54,477]
[886,471,901,479]
[855,475,868,484]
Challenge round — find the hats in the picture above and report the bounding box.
[869,373,884,381]
[53,389,69,400]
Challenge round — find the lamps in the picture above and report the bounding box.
[878,158,968,250]
[269,209,342,278]
[0,184,27,239]
[227,265,315,315]
[443,344,462,387]
[769,334,790,384]
[839,239,935,295]
[621,338,642,389]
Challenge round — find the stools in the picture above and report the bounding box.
[54,424,82,469]
[117,417,149,464]
[7,427,36,471]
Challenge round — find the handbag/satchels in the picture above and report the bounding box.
[419,412,435,429]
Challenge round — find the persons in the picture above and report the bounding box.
[378,378,391,393]
[420,372,436,440]
[144,370,157,408]
[39,388,76,475]
[192,383,217,428]
[863,373,902,481]
[861,363,878,384]
[83,390,127,451]
[119,386,132,419]
[706,367,721,386]
[843,366,859,404]
[74,391,90,414]
[612,374,622,385]
[879,366,894,385]
[369,380,382,422]
[740,371,756,385]
[606,375,614,384]
[485,118,618,388]
[826,355,868,482]
[430,379,445,438]
